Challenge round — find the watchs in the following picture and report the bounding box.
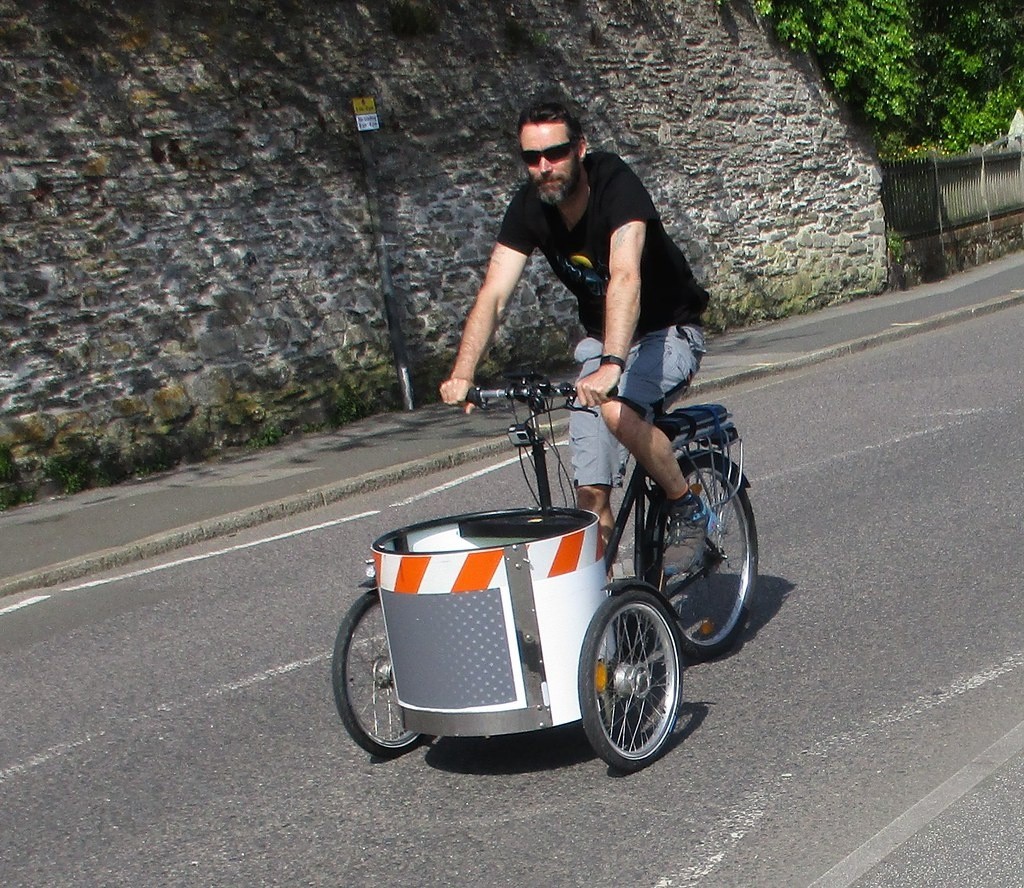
[600,355,626,374]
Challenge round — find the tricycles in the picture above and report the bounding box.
[334,370,759,774]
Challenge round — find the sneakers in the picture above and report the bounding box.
[662,494,717,575]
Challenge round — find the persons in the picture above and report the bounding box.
[439,102,715,573]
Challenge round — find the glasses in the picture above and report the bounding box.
[519,137,579,166]
